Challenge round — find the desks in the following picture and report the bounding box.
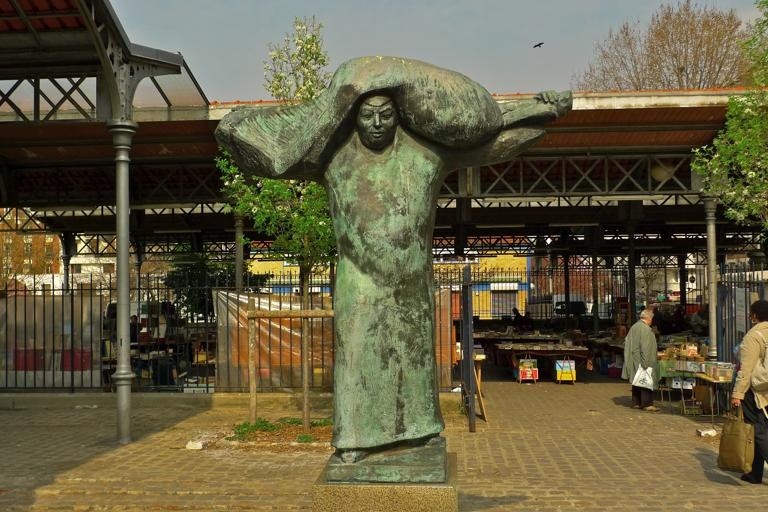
[456,326,735,428]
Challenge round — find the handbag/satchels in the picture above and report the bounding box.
[631,364,654,392]
[716,400,755,474]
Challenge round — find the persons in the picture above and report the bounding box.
[523,311,535,332]
[130,315,143,350]
[637,305,709,337]
[213,54,574,465]
[511,307,524,331]
[619,309,662,412]
[730,299,767,485]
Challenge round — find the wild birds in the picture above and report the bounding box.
[532,41,546,50]
